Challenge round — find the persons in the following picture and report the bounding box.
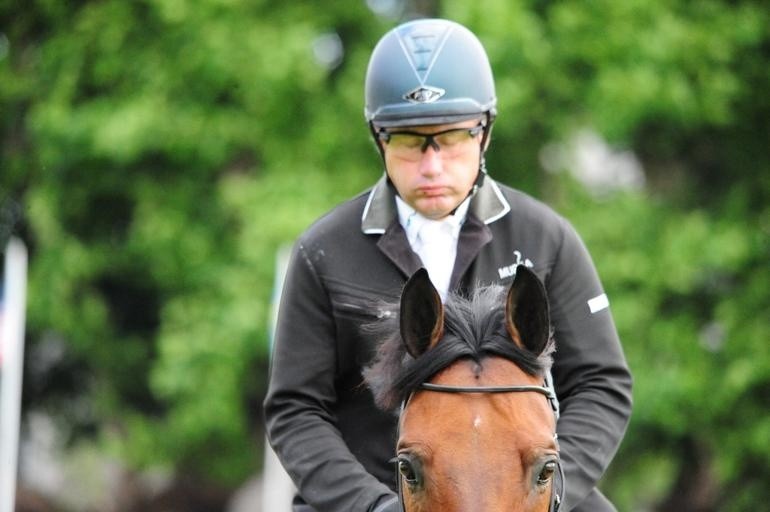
[259,17,635,512]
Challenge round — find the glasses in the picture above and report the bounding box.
[379,125,484,163]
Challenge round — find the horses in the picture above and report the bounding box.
[359,265,559,512]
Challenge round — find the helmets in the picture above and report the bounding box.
[364,19,498,123]
